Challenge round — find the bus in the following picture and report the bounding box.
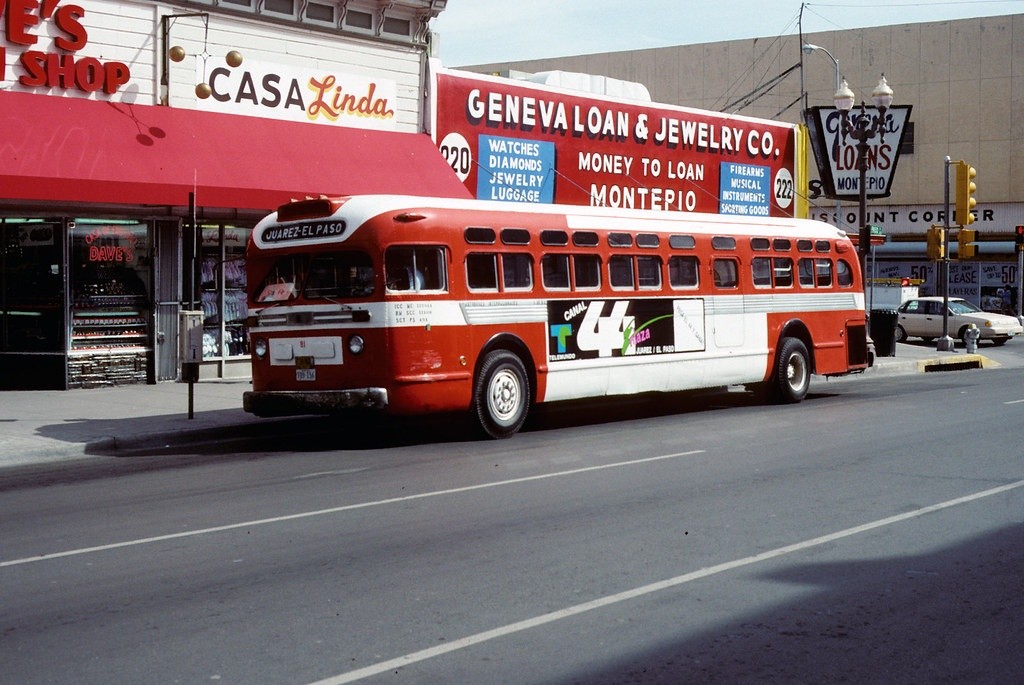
[243,193,868,440]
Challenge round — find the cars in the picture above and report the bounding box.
[893,296,1023,349]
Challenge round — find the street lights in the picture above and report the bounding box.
[803,42,894,367]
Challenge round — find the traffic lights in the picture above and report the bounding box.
[956,160,976,225]
[1015,225,1024,252]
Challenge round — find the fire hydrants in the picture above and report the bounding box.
[964,323,980,355]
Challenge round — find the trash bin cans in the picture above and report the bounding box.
[869,308,899,357]
